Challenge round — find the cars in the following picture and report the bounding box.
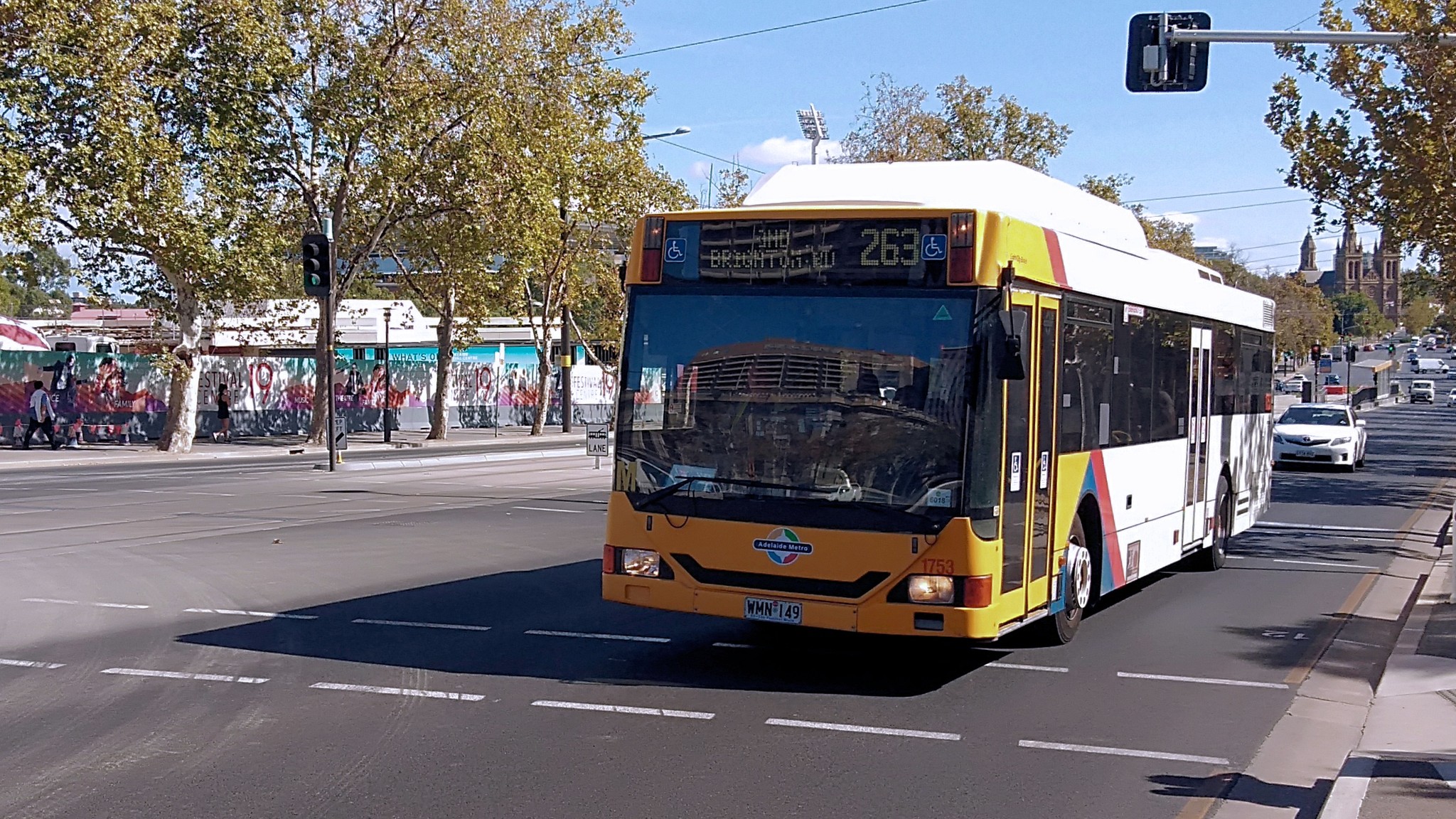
[1382,332,1392,340]
[1407,354,1419,362]
[1363,343,1374,352]
[1272,403,1368,473]
[1408,379,1436,405]
[1325,373,1341,385]
[1284,374,1308,394]
[1410,326,1456,360]
[1407,344,1417,353]
[1446,388,1456,408]
[1373,343,1389,350]
[1274,379,1284,391]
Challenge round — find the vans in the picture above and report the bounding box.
[1319,353,1332,361]
[1410,358,1450,374]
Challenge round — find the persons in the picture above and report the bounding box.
[364,363,412,410]
[509,368,527,406]
[93,356,146,415]
[36,353,79,416]
[1131,362,1176,440]
[212,384,232,445]
[344,361,364,397]
[856,372,927,415]
[22,381,60,451]
[1218,370,1251,414]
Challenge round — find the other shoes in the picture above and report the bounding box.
[23,446,32,450]
[54,441,62,450]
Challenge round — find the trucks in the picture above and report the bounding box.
[1329,346,1343,362]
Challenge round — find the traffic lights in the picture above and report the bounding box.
[1310,344,1321,361]
[1387,344,1394,355]
[301,234,332,298]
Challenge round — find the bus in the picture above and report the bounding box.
[599,101,1278,649]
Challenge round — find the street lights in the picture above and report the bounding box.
[556,125,692,434]
[383,307,392,410]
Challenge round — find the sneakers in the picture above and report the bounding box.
[212,432,218,443]
[224,439,232,444]
